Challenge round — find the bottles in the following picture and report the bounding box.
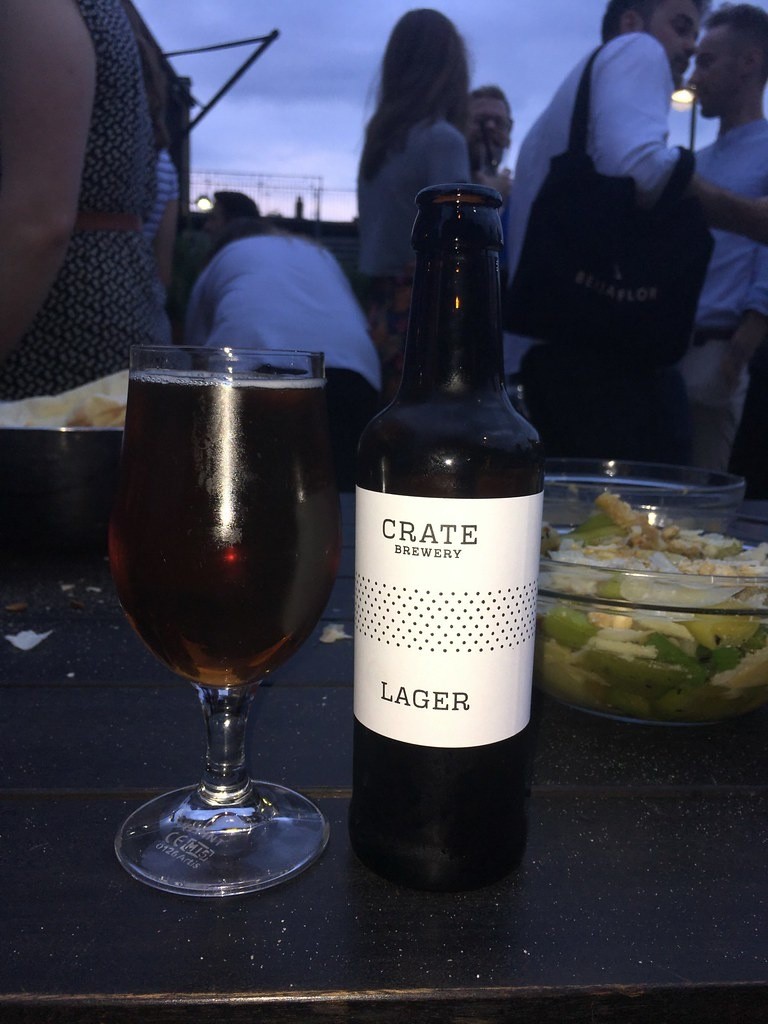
[350,183,546,891]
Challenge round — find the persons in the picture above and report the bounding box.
[0,0,768,580]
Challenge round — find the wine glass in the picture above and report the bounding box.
[106,340,341,898]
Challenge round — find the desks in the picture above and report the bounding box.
[0,496,768,1024]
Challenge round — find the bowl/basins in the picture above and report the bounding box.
[536,455,768,724]
[0,424,124,557]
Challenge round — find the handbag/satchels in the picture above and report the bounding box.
[501,152,717,365]
